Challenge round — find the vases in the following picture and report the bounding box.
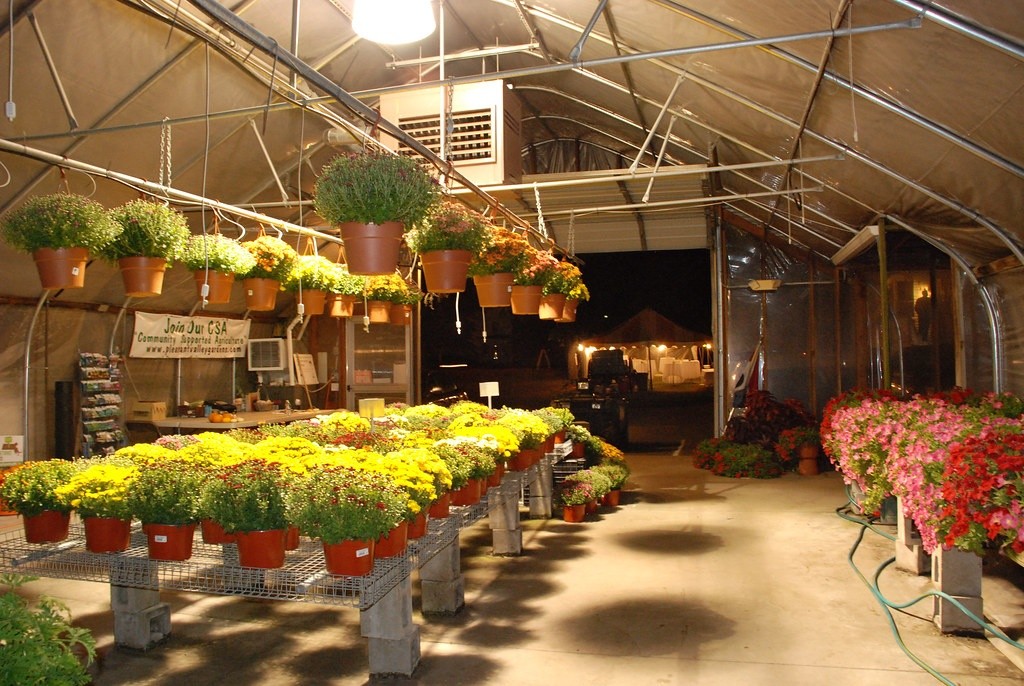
[793,439,821,475]
[413,248,470,293]
[509,284,543,315]
[473,271,517,308]
[366,299,392,322]
[537,294,565,320]
[30,246,89,290]
[116,256,167,297]
[193,268,234,304]
[326,292,355,317]
[241,277,280,312]
[292,288,326,315]
[553,297,579,322]
[335,220,405,277]
[388,303,412,325]
[22,429,620,578]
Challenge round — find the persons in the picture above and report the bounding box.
[914,290,932,341]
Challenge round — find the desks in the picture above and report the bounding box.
[144,408,334,438]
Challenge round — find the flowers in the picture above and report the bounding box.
[308,141,442,231]
[689,426,819,478]
[179,201,588,306]
[0,400,628,545]
[823,380,1023,556]
[0,193,125,255]
[91,198,194,261]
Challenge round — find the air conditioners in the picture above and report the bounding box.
[247,337,287,371]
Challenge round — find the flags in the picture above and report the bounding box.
[731,342,760,408]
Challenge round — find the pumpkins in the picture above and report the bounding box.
[208,410,236,422]
